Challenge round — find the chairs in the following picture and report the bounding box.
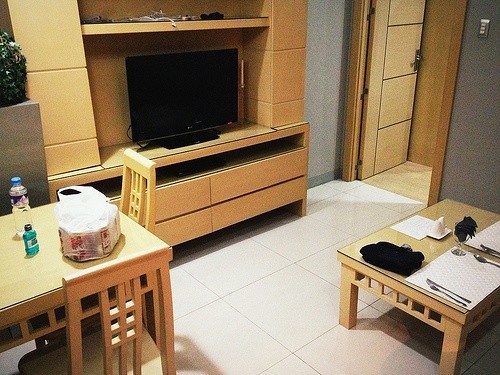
[18,245,173,375]
[119,148,158,236]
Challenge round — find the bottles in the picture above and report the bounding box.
[22,224,39,256]
[8,176,33,239]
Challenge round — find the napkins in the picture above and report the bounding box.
[390,215,435,241]
[404,246,500,311]
[464,220,500,258]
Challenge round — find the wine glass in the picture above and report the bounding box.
[450,221,471,256]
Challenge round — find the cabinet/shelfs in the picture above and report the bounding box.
[6,0,310,248]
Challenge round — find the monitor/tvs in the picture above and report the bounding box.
[125,47,239,150]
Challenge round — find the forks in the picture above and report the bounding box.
[426,280,467,307]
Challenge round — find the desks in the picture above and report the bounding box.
[0,202,176,375]
[336,198,500,375]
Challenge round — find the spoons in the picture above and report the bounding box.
[473,254,500,268]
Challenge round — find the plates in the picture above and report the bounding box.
[426,227,452,240]
